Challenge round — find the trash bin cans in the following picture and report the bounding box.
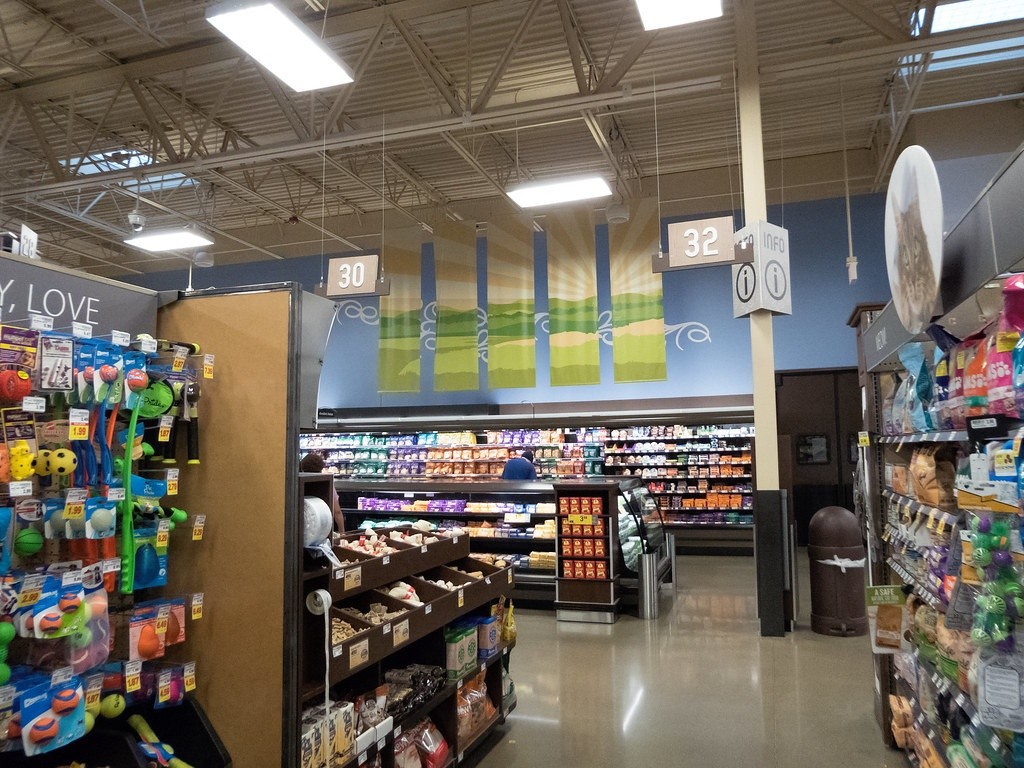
[807,506,868,637]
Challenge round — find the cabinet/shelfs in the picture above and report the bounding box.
[331,475,678,624]
[856,144,1024,768]
[297,470,516,768]
[299,393,755,555]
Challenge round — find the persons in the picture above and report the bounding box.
[502,451,537,480]
[300,452,344,532]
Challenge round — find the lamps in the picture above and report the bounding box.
[505,108,613,208]
[205,0,356,93]
[634,0,723,31]
[122,178,214,253]
